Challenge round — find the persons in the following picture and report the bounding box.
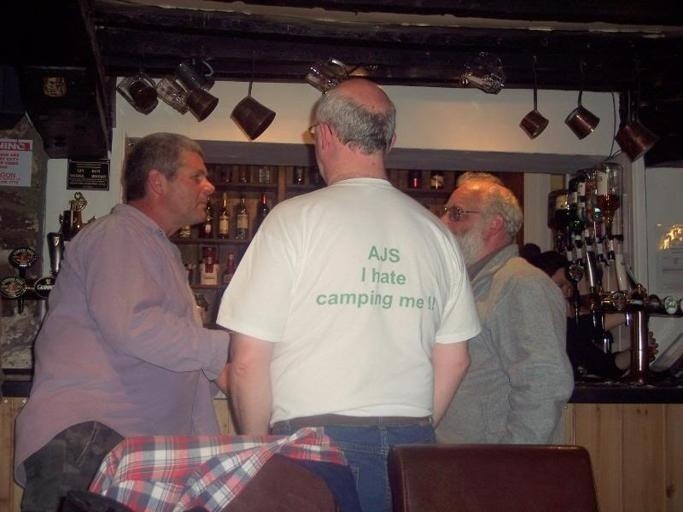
[216,77,482,511]
[528,249,659,384]
[432,169,575,446]
[11,133,230,512]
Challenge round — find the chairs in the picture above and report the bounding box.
[88,435,363,512]
[387,444,600,511]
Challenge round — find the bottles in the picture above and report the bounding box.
[630,310,648,386]
[178,164,271,286]
[388,170,444,188]
[295,167,322,184]
[547,163,683,313]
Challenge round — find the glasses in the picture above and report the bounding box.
[307,122,332,138]
[435,205,481,220]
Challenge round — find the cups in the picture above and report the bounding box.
[459,52,507,95]
[155,72,192,115]
[117,69,158,115]
[613,117,653,162]
[183,87,219,122]
[174,57,221,92]
[302,56,349,95]
[518,110,549,140]
[230,95,276,141]
[564,105,601,142]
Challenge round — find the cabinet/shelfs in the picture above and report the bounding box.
[123,164,525,329]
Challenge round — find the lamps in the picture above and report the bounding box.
[113,55,218,123]
[299,57,361,93]
[521,54,549,137]
[614,91,659,164]
[566,57,600,139]
[447,37,507,94]
[230,51,276,140]
